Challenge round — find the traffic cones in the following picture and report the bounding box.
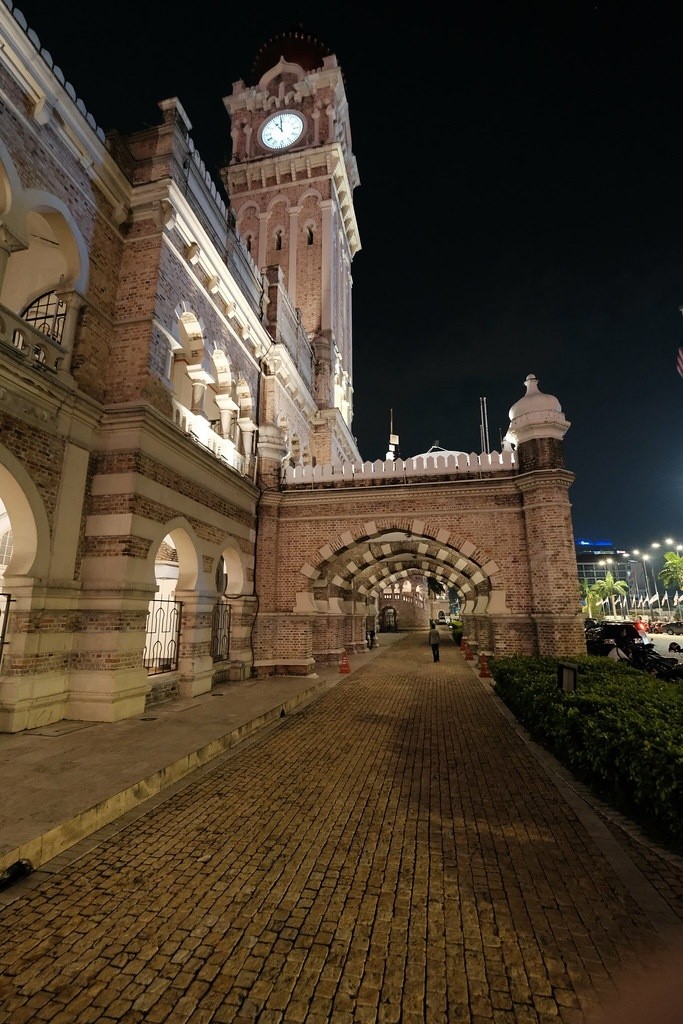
[478,653,492,678]
[339,652,352,674]
[464,645,473,660]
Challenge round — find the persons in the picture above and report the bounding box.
[607,641,630,664]
[429,619,440,662]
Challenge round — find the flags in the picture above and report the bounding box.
[649,593,658,604]
[596,597,608,606]
[674,594,683,606]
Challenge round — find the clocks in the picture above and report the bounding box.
[256,109,307,152]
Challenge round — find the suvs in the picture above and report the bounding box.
[585,620,651,657]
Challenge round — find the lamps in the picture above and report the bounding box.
[556,661,578,693]
[255,346,263,358]
[188,245,201,264]
[225,302,237,319]
[164,210,176,230]
[209,277,220,294]
[33,97,52,124]
[241,327,250,340]
[114,204,131,224]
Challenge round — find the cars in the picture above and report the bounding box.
[438,615,447,625]
[652,622,683,635]
